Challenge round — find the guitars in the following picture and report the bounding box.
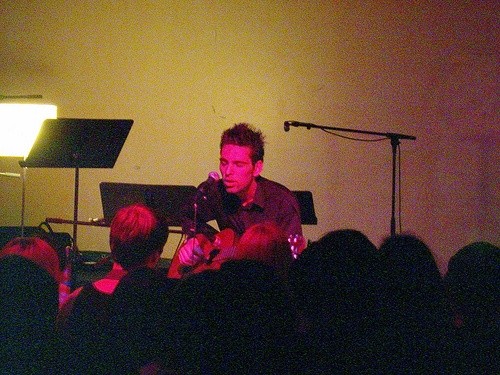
[164,227,307,278]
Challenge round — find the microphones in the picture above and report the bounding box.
[194,170,220,200]
[283,121,290,131]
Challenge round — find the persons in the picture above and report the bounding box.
[178,123,303,268]
[59,203,169,311]
[0,229,500,375]
